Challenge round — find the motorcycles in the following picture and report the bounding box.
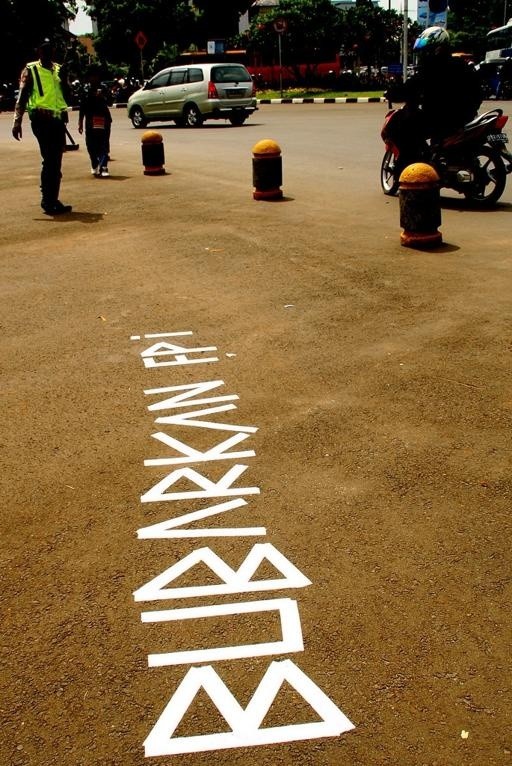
[0,73,145,108]
[480,77,512,100]
[380,74,512,208]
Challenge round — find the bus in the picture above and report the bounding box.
[483,20,512,63]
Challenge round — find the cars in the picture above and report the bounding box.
[327,60,416,80]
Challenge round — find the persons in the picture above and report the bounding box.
[12,29,74,214]
[382,25,484,169]
[78,69,118,178]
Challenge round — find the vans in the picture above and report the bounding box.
[482,57,509,73]
[125,60,261,127]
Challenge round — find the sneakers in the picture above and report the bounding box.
[39,198,73,215]
[90,161,111,177]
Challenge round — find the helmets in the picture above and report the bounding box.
[412,25,452,54]
